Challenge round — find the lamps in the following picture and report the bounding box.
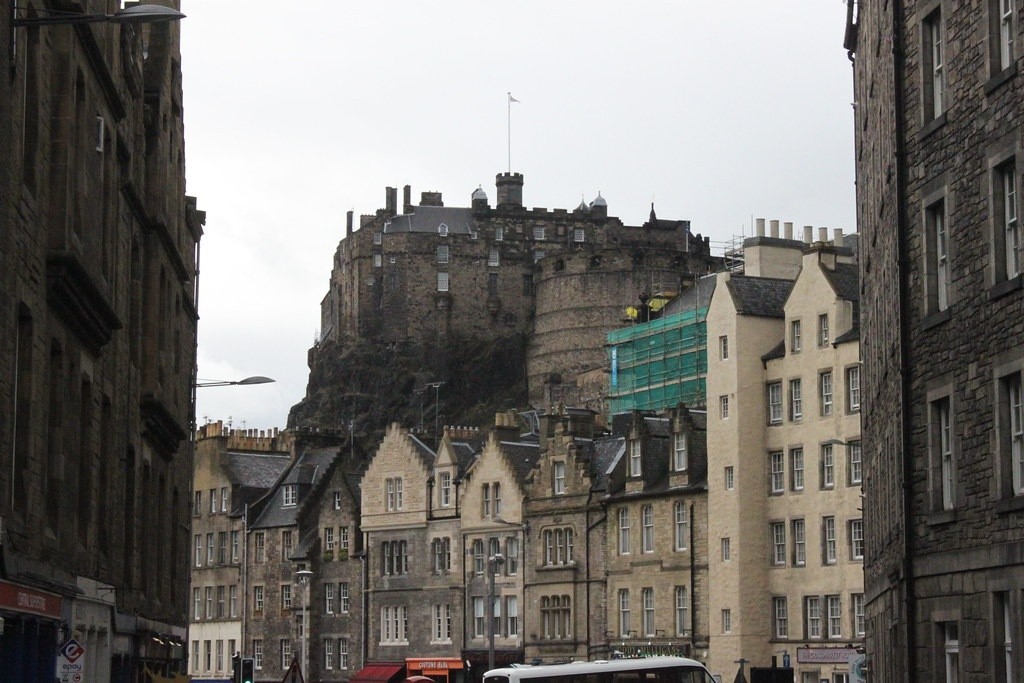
[153,632,183,646]
[9,3,187,27]
[192,376,275,387]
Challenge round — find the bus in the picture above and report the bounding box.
[482,656,717,683]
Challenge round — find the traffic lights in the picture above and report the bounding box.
[242,657,254,683]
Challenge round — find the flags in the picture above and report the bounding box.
[510,96,520,103]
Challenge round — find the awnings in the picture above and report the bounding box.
[349,663,405,683]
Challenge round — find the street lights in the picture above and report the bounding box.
[488,553,506,671]
[298,569,315,682]
